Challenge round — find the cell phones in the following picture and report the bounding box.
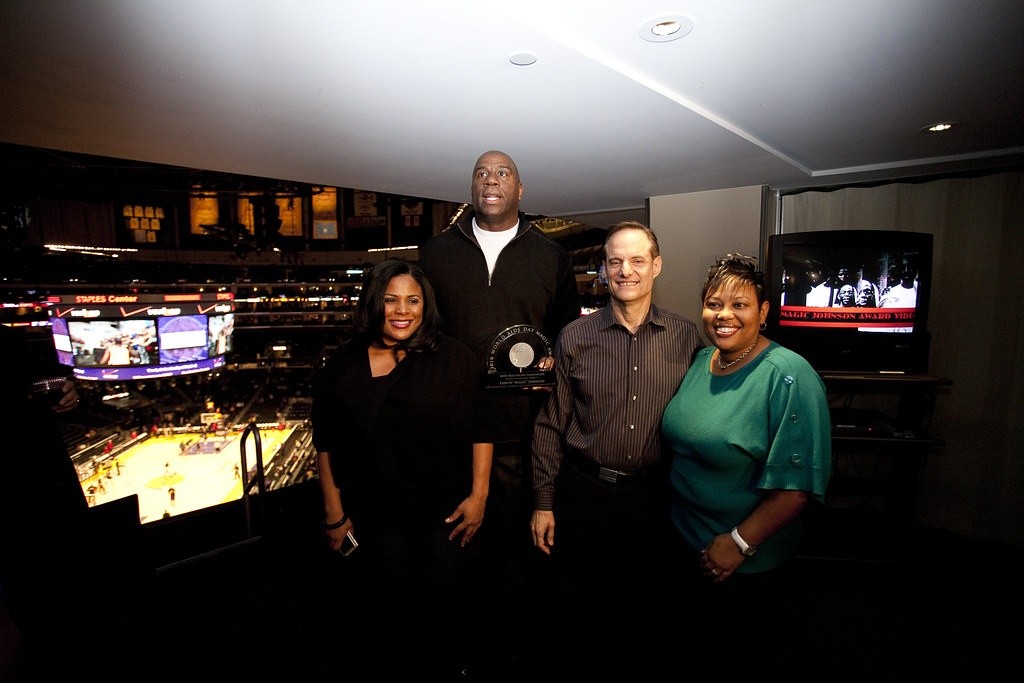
[339,531,358,557]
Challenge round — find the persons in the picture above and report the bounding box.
[83,420,245,514]
[832,249,919,309]
[215,328,227,355]
[307,256,494,550]
[526,219,706,555]
[417,149,579,467]
[658,251,831,584]
[96,334,138,367]
[0,322,101,416]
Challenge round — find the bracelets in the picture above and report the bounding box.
[323,512,347,530]
[731,528,755,557]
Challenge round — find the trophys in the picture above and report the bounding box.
[484,323,558,395]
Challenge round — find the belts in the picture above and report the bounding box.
[583,461,653,488]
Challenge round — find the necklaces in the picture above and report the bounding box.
[718,338,757,368]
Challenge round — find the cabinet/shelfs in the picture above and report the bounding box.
[793,371,954,586]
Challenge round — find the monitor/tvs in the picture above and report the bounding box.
[767,229,933,375]
[50,314,237,368]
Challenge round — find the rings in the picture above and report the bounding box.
[74,397,80,402]
[711,567,722,577]
[73,392,76,397]
